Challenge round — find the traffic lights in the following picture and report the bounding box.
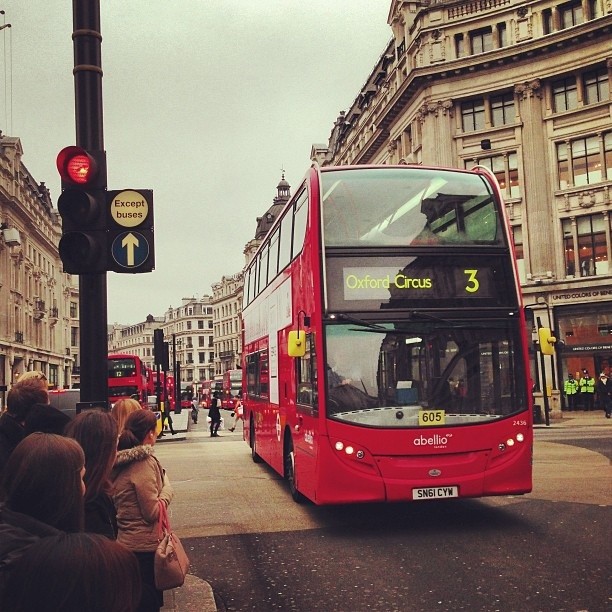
[56,146,98,274]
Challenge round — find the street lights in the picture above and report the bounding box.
[530,316,550,426]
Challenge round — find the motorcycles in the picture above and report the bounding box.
[191,409,198,423]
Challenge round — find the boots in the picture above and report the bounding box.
[228,427,235,432]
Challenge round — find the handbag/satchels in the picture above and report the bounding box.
[238,405,243,415]
[191,412,197,418]
[207,416,225,432]
[154,499,190,591]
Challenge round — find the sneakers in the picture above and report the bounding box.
[160,434,166,436]
[214,433,219,436]
[211,433,214,436]
[606,412,610,418]
[172,431,177,435]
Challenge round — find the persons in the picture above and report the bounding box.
[579,369,595,411]
[110,399,141,445]
[60,409,118,543]
[189,396,200,425]
[597,371,612,418]
[563,373,579,413]
[162,392,177,435]
[109,410,174,612]
[16,370,53,405]
[327,347,374,412]
[228,395,244,435]
[207,390,220,437]
[0,386,48,466]
[0,430,138,609]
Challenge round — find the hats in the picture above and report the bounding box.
[583,369,589,373]
[600,373,605,376]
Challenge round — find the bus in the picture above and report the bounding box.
[241,161,556,504]
[180,382,194,408]
[167,375,175,411]
[106,353,148,411]
[147,368,154,396]
[222,370,242,410]
[202,380,223,409]
[152,371,166,411]
